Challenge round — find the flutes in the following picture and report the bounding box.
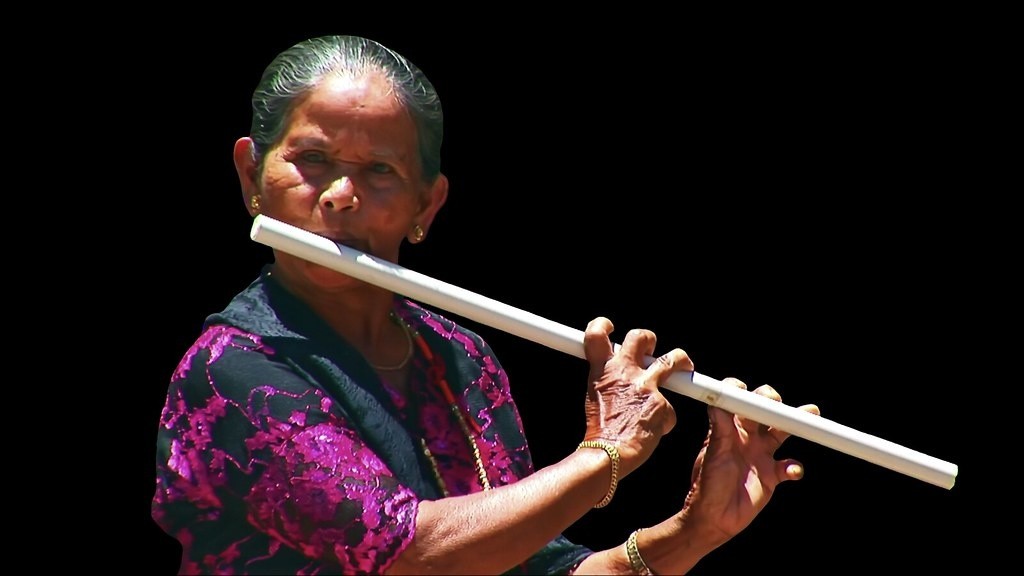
[247,212,962,496]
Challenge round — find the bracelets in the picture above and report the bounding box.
[627,528,655,576]
[576,441,620,509]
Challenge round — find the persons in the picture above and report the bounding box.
[152,36,822,576]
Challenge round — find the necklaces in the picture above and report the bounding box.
[359,308,490,498]
